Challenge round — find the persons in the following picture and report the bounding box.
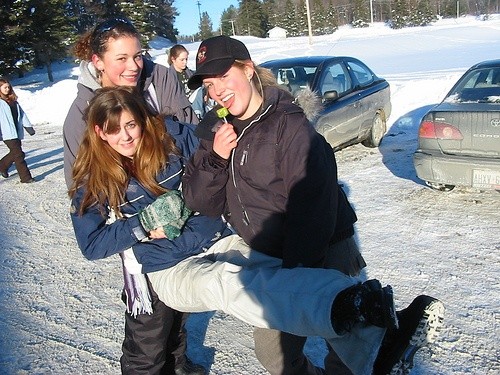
[0,78,37,184]
[181,35,367,375]
[68,87,444,375]
[167,44,196,99]
[62,18,211,375]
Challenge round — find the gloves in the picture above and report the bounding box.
[139,190,193,241]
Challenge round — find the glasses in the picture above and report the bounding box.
[91,16,131,43]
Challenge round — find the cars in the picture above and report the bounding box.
[253,55,392,152]
[411,60,500,193]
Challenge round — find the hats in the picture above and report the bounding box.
[187,36,251,90]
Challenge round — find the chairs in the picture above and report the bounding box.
[278,70,300,95]
[321,71,340,96]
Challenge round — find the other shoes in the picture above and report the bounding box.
[1,171,8,178]
[332,279,399,336]
[373,295,445,375]
[22,177,36,183]
[168,354,206,375]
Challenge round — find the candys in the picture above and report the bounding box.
[216,107,229,118]
